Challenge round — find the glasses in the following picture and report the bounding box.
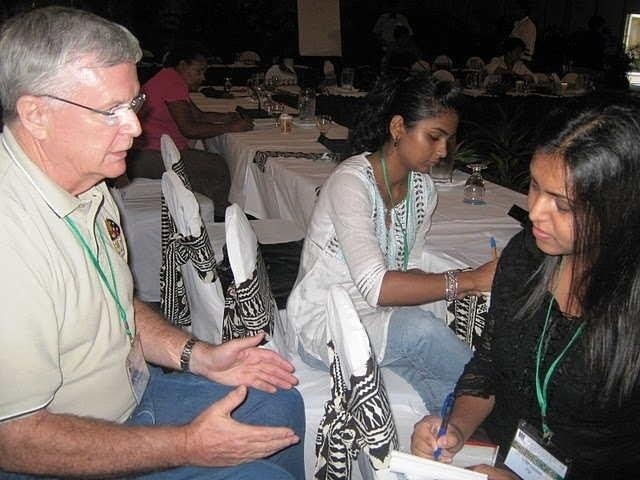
[38,93,147,126]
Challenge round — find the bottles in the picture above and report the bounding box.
[264,93,272,118]
[224,74,232,96]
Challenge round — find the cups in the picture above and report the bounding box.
[341,72,352,91]
[567,55,575,74]
[298,87,316,124]
[515,80,525,91]
[324,60,337,88]
[431,136,455,183]
[279,116,293,136]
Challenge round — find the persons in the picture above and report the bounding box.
[372,2,414,49]
[536,11,614,76]
[388,26,422,65]
[285,75,498,416]
[485,38,534,82]
[127,40,254,221]
[0,6,305,480]
[509,1,538,63]
[410,100,640,480]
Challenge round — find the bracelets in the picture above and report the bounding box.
[178,336,200,376]
[440,268,459,302]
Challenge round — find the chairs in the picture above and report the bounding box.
[161,171,286,345]
[225,203,429,478]
[110,189,215,304]
[313,285,399,480]
[117,179,163,192]
[159,133,305,262]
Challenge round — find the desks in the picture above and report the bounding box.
[272,78,589,125]
[205,64,264,87]
[180,86,530,353]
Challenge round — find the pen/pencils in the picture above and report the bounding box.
[239,111,245,120]
[490,236,496,259]
[432,392,456,460]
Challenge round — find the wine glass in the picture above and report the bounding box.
[272,104,283,128]
[316,115,332,138]
[463,164,488,205]
